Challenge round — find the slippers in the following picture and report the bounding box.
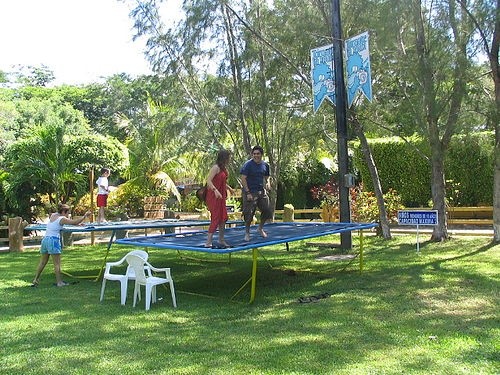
[298,292,330,303]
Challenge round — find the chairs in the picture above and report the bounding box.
[126,254,177,311]
[100,250,149,305]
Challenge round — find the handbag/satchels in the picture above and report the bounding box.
[196,187,207,202]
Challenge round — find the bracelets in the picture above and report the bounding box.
[246,191,251,195]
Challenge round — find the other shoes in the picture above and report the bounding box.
[57,281,69,287]
[31,285,39,287]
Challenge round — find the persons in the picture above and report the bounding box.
[33,204,91,287]
[196,151,236,249]
[241,146,273,242]
[96,167,110,222]
